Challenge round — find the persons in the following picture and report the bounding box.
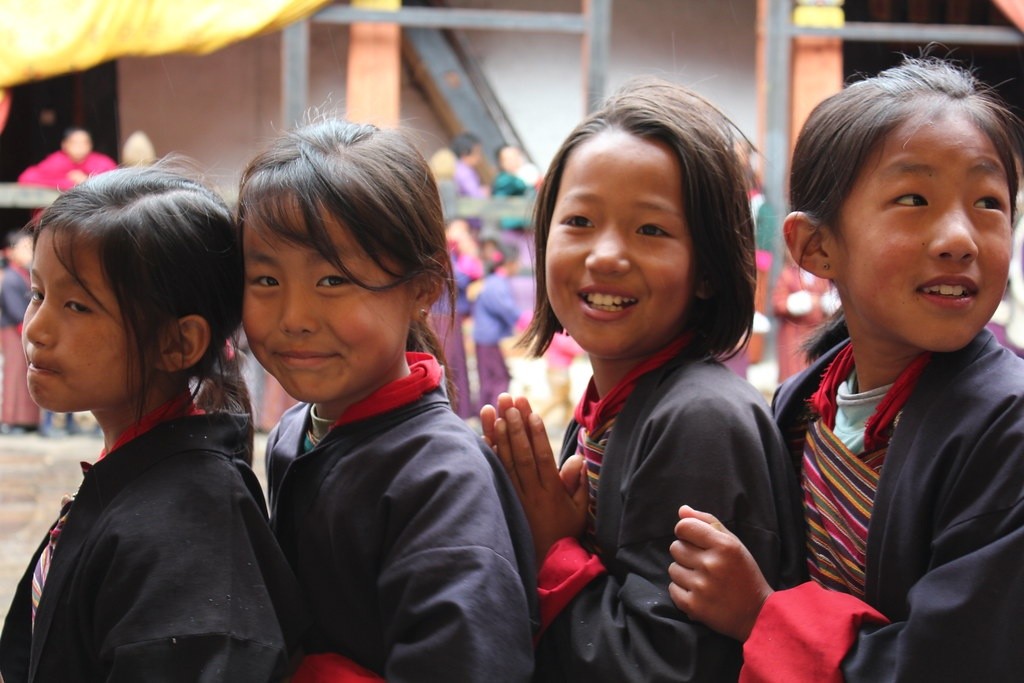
[473,82,812,683]
[229,117,545,683]
[423,130,837,425]
[664,53,1023,683]
[0,151,303,683]
[18,124,122,227]
[0,227,105,439]
